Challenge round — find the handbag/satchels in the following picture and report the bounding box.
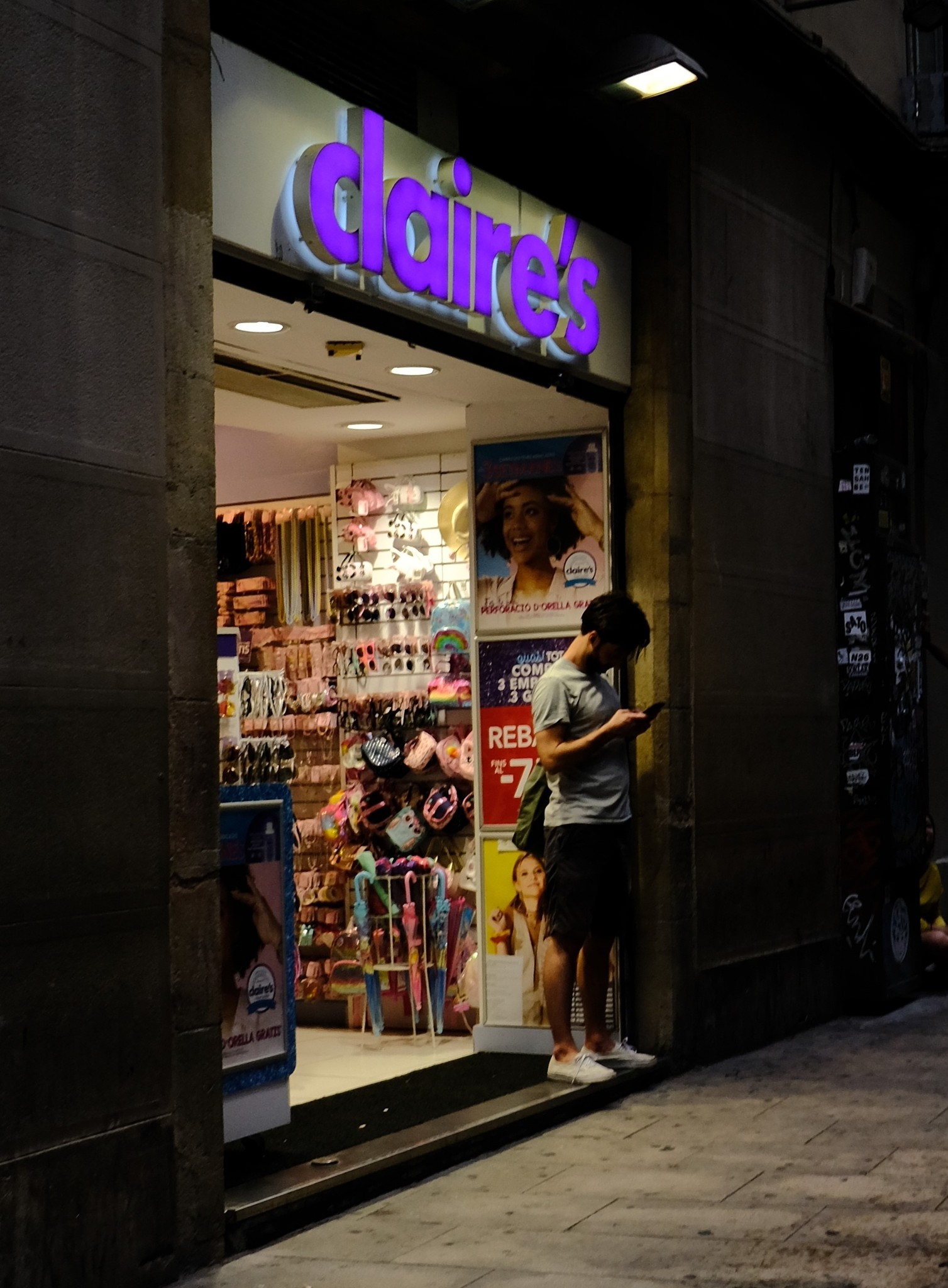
[357,785,393,831]
[384,806,427,852]
[319,784,363,842]
[401,729,436,774]
[436,734,461,779]
[360,731,403,772]
[422,782,458,830]
[425,658,472,707]
[509,754,552,856]
[331,473,437,584]
[455,729,475,782]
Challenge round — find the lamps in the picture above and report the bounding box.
[597,34,709,104]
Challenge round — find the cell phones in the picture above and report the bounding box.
[642,702,666,719]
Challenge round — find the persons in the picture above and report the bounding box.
[490,859,557,1028]
[474,470,608,608]
[529,595,658,1086]
[217,842,286,1059]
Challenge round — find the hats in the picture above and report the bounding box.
[437,480,471,560]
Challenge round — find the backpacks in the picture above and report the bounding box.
[428,582,473,655]
[328,920,366,996]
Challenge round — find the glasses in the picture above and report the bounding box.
[217,669,297,785]
[322,582,437,731]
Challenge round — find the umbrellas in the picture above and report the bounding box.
[352,871,475,1035]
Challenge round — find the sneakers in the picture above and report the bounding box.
[581,1037,658,1068]
[546,1051,617,1084]
